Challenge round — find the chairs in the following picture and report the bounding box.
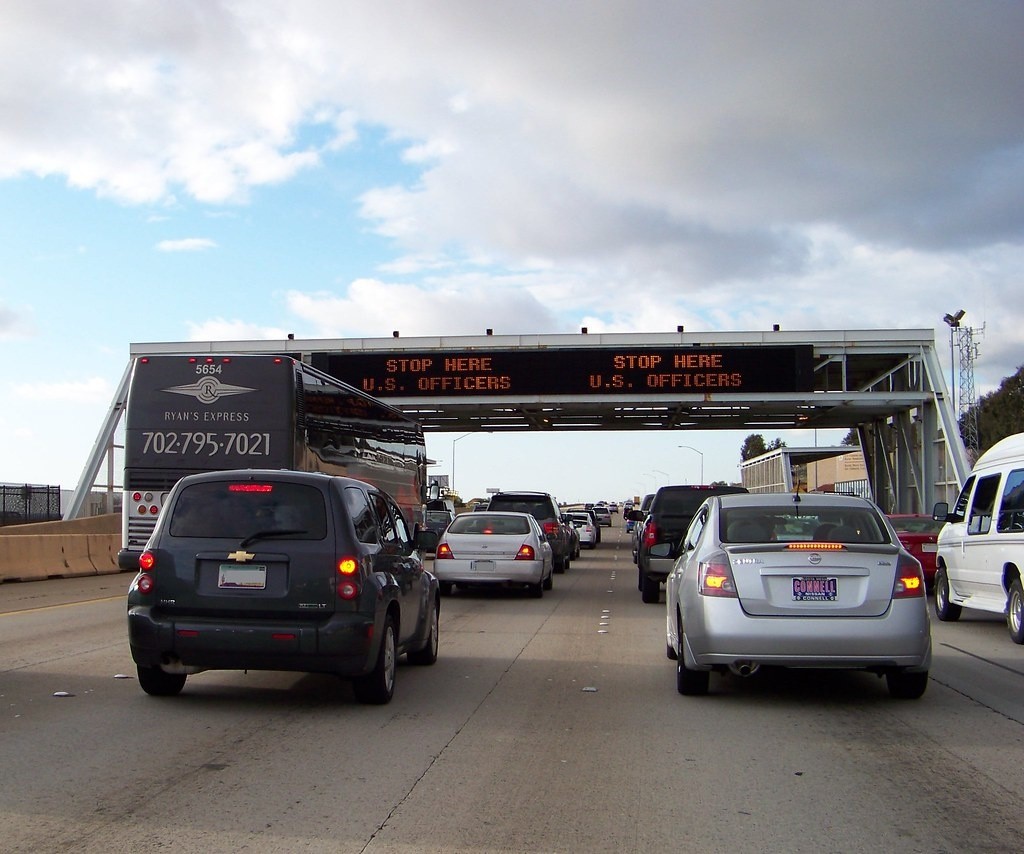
[813,524,836,540]
[727,520,769,542]
[827,526,858,542]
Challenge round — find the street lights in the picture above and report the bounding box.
[643,471,669,488]
[942,308,966,413]
[678,445,703,485]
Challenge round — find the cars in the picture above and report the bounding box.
[434,513,554,596]
[884,512,946,590]
[623,505,632,519]
[562,513,597,548]
[568,521,582,560]
[627,519,634,533]
[585,503,593,509]
[647,491,930,701]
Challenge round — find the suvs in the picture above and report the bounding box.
[566,508,603,542]
[127,471,440,702]
[626,486,751,604]
[487,490,575,574]
[592,508,613,527]
[632,493,657,563]
[609,504,619,514]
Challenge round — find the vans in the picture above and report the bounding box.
[932,432,1024,643]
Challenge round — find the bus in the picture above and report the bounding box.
[117,355,440,577]
